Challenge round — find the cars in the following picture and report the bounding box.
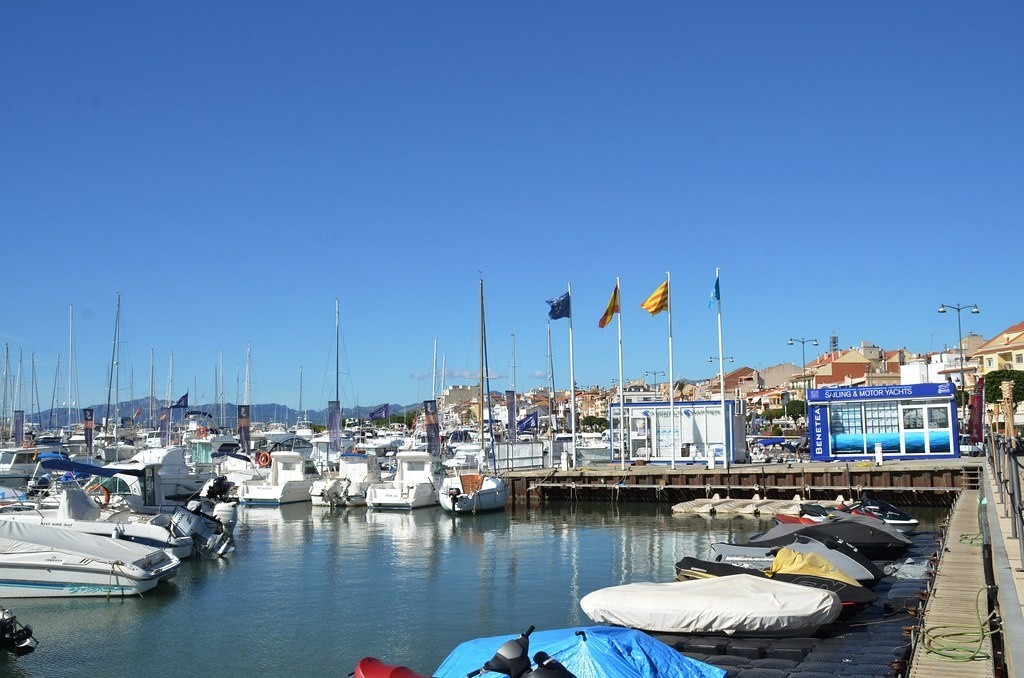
[958,433,982,458]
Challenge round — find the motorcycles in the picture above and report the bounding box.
[673,543,879,612]
[773,503,914,555]
[796,498,921,533]
[345,623,580,677]
[710,529,888,585]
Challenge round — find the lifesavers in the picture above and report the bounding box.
[85,485,109,510]
[198,426,208,438]
[258,450,270,466]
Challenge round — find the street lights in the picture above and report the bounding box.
[643,370,666,400]
[787,337,819,436]
[938,302,980,434]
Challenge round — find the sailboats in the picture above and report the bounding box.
[1,267,638,595]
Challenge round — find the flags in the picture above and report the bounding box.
[13,410,24,448]
[640,280,668,316]
[171,391,188,409]
[598,283,619,328]
[422,400,440,455]
[708,276,720,307]
[518,410,538,432]
[968,378,982,444]
[160,406,170,448]
[133,405,141,418]
[237,404,251,455]
[544,291,569,320]
[505,390,516,439]
[328,401,342,451]
[368,403,389,420]
[82,409,95,455]
